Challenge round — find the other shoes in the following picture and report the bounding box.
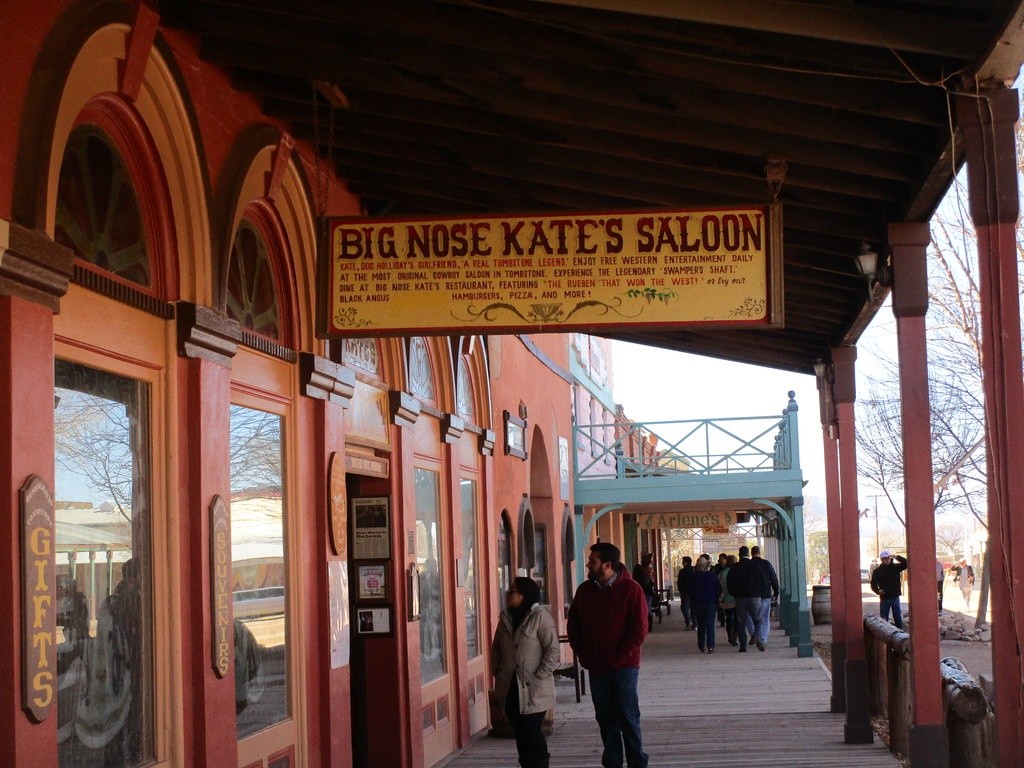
[749,635,757,645]
[699,647,705,652]
[938,609,942,616]
[756,641,765,652]
[728,640,739,646]
[708,648,717,654]
[739,649,746,652]
[693,627,697,631]
[721,623,725,627]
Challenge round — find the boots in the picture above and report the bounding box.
[518,753,550,768]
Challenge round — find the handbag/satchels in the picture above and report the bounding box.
[735,589,754,610]
[719,591,737,609]
[244,632,263,702]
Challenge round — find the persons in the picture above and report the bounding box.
[871,550,908,630]
[630,545,779,655]
[419,559,444,662]
[53,557,145,768]
[818,573,830,584]
[935,555,945,616]
[870,559,879,585]
[232,618,261,716]
[566,541,649,768]
[950,558,975,614]
[490,576,561,768]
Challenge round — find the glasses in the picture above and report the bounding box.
[506,590,519,595]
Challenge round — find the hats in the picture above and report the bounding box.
[880,551,890,558]
